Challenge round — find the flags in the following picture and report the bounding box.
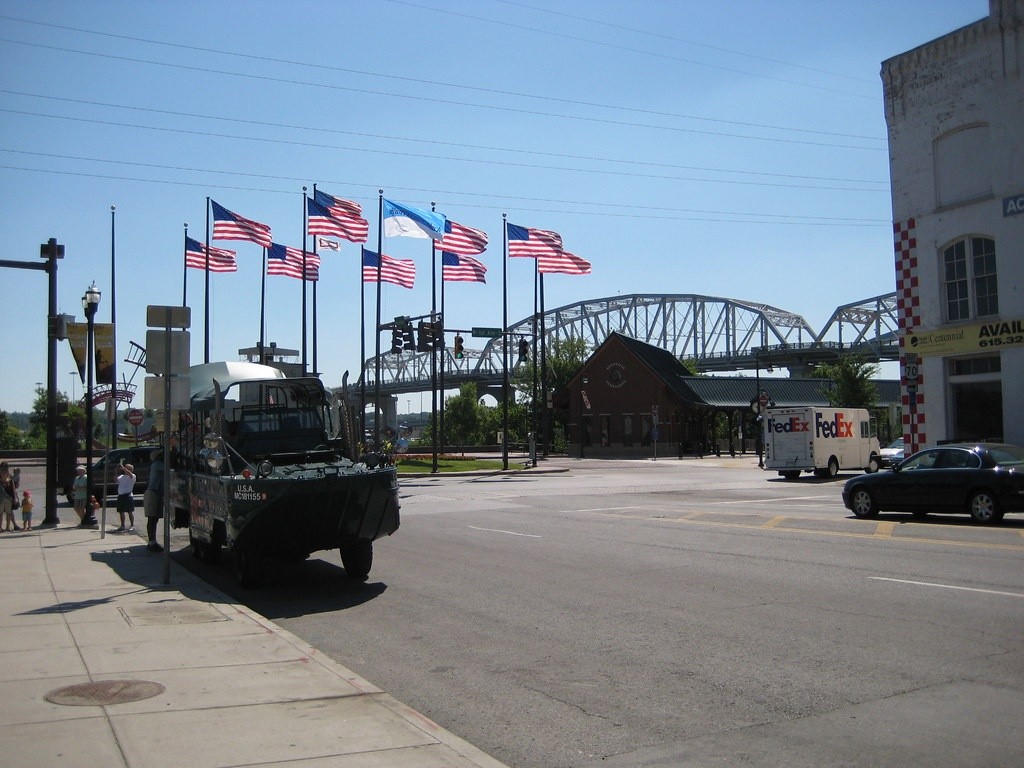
[211,199,272,251]
[433,219,488,255]
[441,250,488,284]
[186,236,238,273]
[316,237,342,252]
[362,248,415,289]
[382,197,446,241]
[316,191,362,217]
[538,252,592,274]
[506,222,563,259]
[266,242,321,281]
[306,196,369,244]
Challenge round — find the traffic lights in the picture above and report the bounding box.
[401,324,416,350]
[518,339,528,362]
[392,326,402,355]
[417,321,433,352]
[429,320,445,348]
[454,336,463,359]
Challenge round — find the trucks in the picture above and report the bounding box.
[170,361,415,593]
[761,406,882,480]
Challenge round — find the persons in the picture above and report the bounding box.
[20,491,33,531]
[114,463,137,531]
[0,462,20,532]
[71,465,88,526]
[143,449,165,553]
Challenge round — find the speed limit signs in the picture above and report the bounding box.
[904,362,919,381]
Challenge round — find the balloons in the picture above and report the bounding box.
[91,495,100,509]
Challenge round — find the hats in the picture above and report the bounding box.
[76,465,86,472]
[150,450,162,461]
[23,491,31,496]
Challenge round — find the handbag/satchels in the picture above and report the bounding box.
[11,496,20,510]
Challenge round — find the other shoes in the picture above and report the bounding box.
[0,529,5,533]
[5,527,12,531]
[115,527,126,532]
[129,526,136,531]
[24,528,28,530]
[28,527,32,531]
[14,525,21,530]
[148,542,163,552]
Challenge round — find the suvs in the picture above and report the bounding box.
[64,444,161,506]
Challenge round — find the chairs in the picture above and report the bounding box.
[227,421,255,435]
[280,416,300,429]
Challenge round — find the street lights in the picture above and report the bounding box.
[77,281,102,529]
[755,348,774,468]
[69,371,79,403]
[578,362,591,458]
[36,381,43,401]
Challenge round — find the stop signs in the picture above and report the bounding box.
[128,409,144,426]
[651,404,659,417]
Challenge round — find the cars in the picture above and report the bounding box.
[842,442,1024,526]
[880,437,905,468]
